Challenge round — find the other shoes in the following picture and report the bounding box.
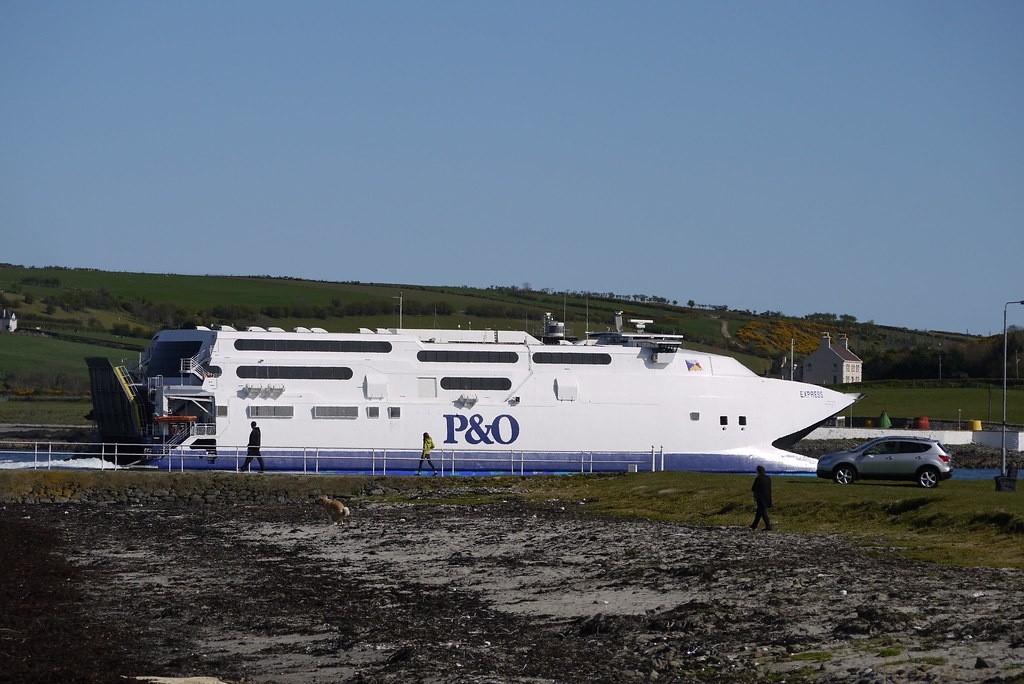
[432,471,438,476]
[414,473,420,476]
[258,470,265,473]
[749,525,754,530]
[236,469,243,472]
[761,528,772,531]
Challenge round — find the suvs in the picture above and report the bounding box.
[817,435,953,488]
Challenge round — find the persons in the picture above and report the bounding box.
[238,421,266,472]
[749,465,773,532]
[414,433,438,476]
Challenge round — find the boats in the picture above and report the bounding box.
[82,292,873,476]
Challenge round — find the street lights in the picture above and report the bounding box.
[1001,300,1024,476]
